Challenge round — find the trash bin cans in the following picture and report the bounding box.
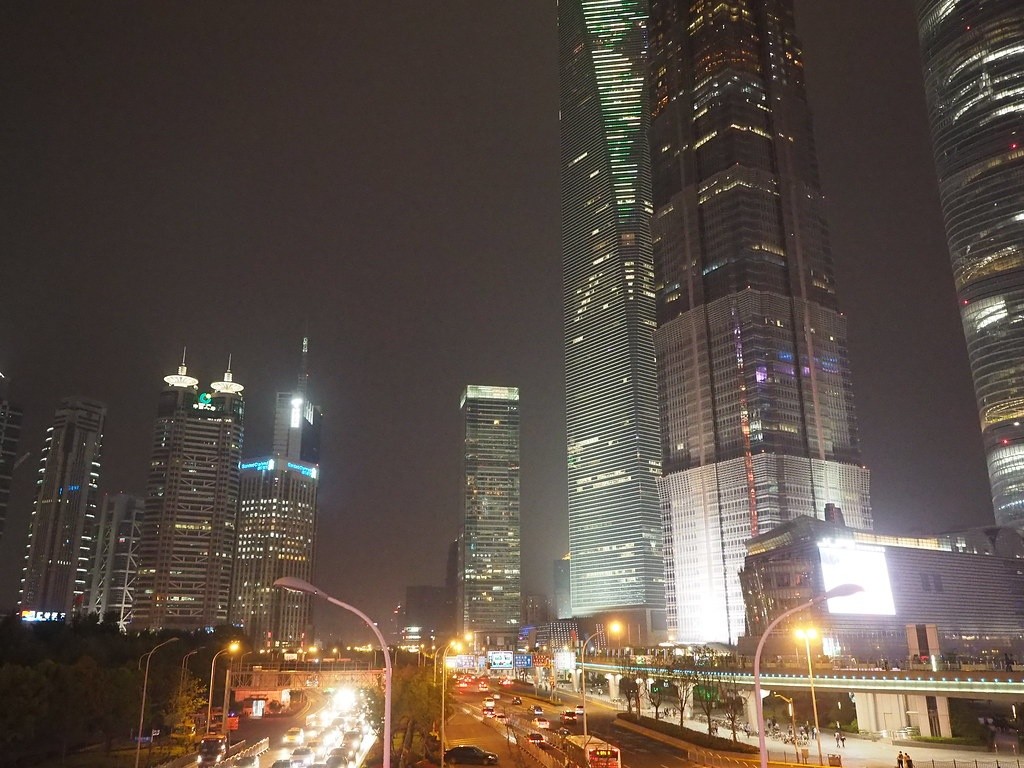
[827,753,843,768]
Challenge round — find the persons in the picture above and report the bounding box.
[834,730,847,748]
[744,721,752,739]
[896,750,914,768]
[708,720,720,736]
[664,707,677,718]
[766,715,817,739]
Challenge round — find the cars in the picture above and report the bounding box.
[443,745,499,766]
[511,696,522,705]
[455,675,488,695]
[480,693,508,725]
[232,755,258,768]
[576,705,584,715]
[271,715,376,768]
[527,704,549,729]
[525,731,545,749]
[553,728,572,740]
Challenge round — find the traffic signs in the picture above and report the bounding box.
[513,654,532,669]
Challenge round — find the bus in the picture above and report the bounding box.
[563,735,621,768]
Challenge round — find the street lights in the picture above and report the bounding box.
[434,632,473,768]
[135,638,177,768]
[274,577,390,768]
[208,643,238,735]
[796,628,823,766]
[179,646,205,718]
[755,584,863,768]
[582,622,622,768]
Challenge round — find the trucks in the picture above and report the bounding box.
[197,735,246,768]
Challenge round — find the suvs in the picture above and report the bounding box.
[560,710,576,725]
